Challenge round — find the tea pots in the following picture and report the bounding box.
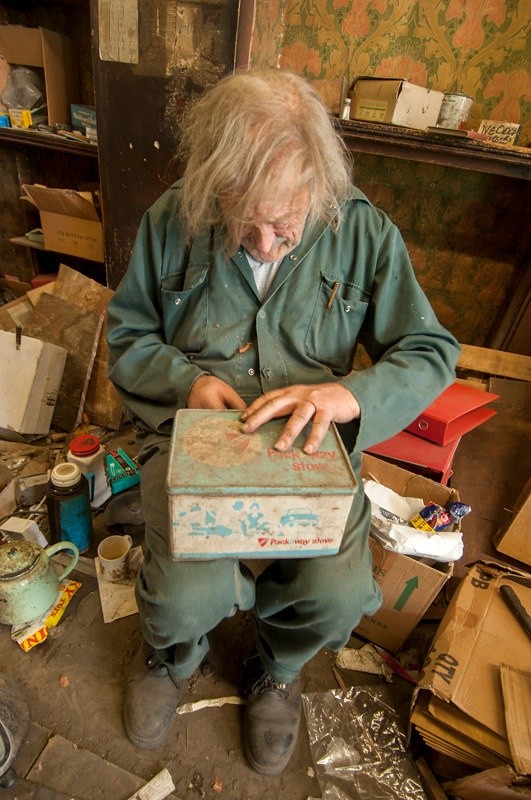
[0,542,79,625]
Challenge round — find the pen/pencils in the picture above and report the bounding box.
[327,283,339,308]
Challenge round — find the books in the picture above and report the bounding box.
[0,263,115,434]
[427,126,489,141]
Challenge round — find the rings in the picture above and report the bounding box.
[303,398,318,413]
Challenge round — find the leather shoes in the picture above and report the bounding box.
[242,667,301,776]
[123,647,187,750]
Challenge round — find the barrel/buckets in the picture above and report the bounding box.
[436,94,475,130]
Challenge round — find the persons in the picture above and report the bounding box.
[121,69,461,775]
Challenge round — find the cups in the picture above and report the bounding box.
[98,535,133,582]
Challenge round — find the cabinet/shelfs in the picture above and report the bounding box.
[0,128,108,289]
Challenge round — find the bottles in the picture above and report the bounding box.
[339,98,351,120]
[45,463,95,556]
[67,435,112,508]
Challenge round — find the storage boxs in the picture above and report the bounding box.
[20,181,104,262]
[167,408,358,560]
[0,331,67,434]
[350,74,446,131]
[0,25,78,126]
[351,452,461,655]
